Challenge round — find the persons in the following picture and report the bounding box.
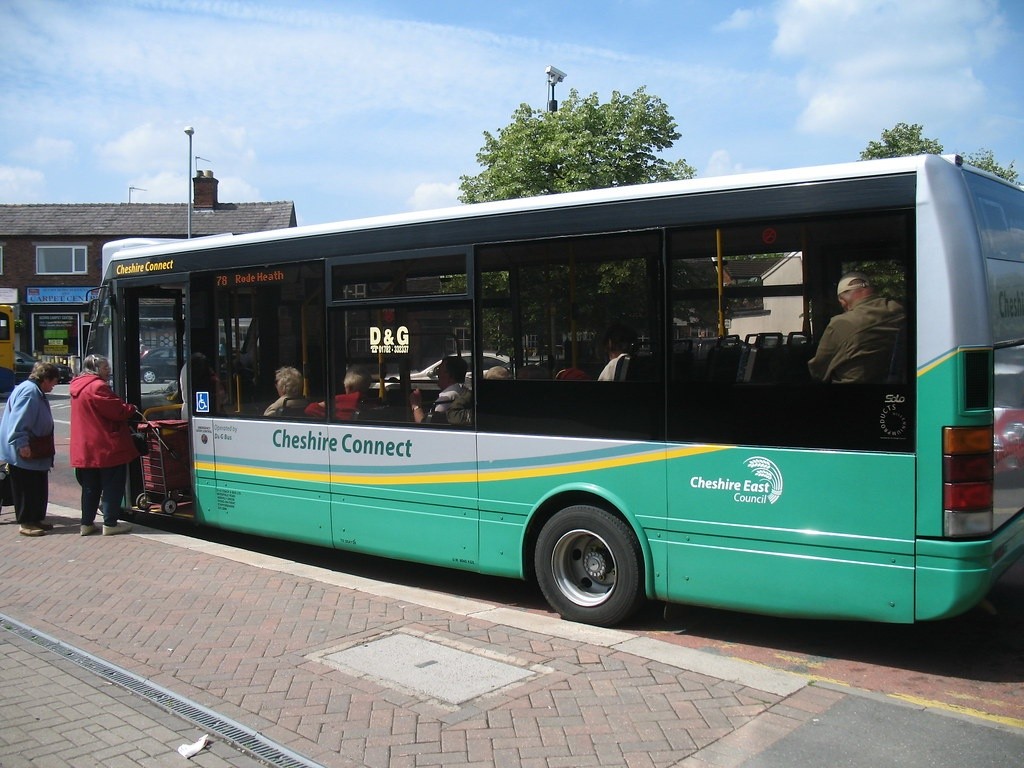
[240,279,300,400]
[408,356,468,423]
[219,337,227,367]
[304,364,385,421]
[0,362,60,535]
[597,323,641,380]
[555,368,591,381]
[446,366,513,425]
[808,270,906,383]
[69,353,140,536]
[263,366,309,416]
[180,359,189,421]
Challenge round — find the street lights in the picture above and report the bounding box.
[183,126,194,238]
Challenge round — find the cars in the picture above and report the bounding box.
[125,344,186,384]
[15,350,74,385]
[368,350,534,391]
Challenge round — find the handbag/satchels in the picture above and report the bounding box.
[0,460,15,506]
[20,434,54,459]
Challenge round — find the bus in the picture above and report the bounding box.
[82,152,1024,629]
[0,304,16,393]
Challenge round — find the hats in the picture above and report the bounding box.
[836,272,871,295]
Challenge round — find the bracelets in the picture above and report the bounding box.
[412,405,422,412]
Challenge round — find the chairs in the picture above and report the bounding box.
[613,329,812,387]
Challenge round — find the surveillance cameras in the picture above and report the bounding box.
[545,66,568,82]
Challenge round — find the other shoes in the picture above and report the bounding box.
[19,522,44,536]
[80,524,98,535]
[102,523,133,535]
[37,521,53,530]
